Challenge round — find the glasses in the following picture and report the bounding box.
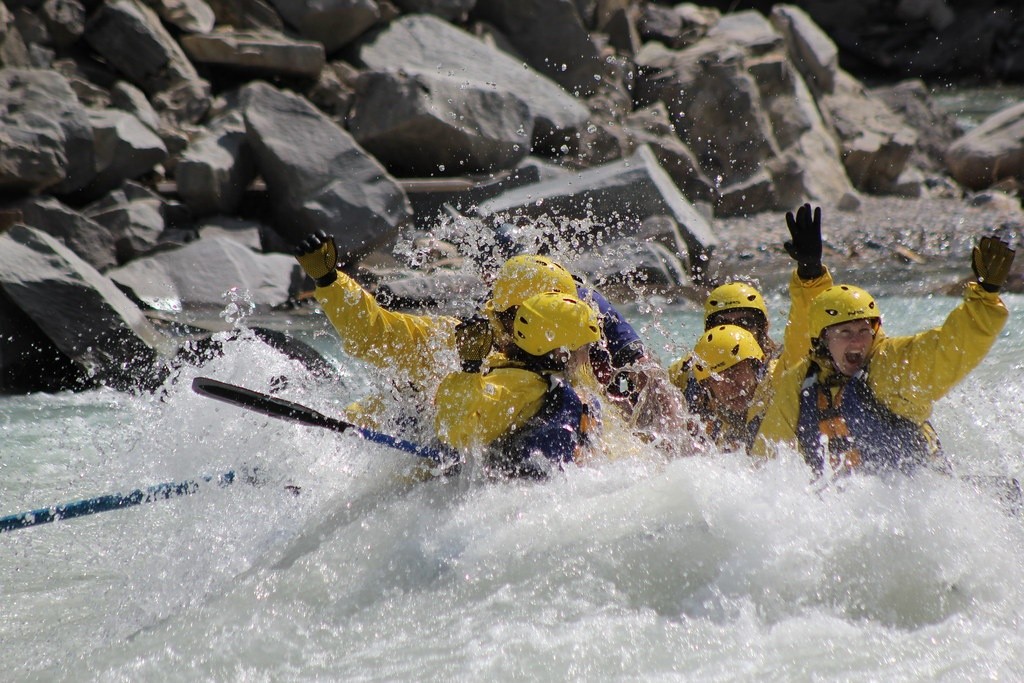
[715,317,763,329]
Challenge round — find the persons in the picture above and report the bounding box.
[292,229,642,469]
[430,292,601,484]
[668,203,833,452]
[749,235,1016,482]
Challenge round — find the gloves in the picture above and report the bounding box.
[294,229,339,286]
[783,203,825,279]
[972,235,1016,292]
[456,313,494,371]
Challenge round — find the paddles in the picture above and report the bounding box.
[0,459,298,531]
[193,374,459,472]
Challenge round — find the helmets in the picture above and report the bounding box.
[691,324,764,382]
[512,292,600,356]
[494,255,578,311]
[811,284,880,337]
[704,281,769,326]
